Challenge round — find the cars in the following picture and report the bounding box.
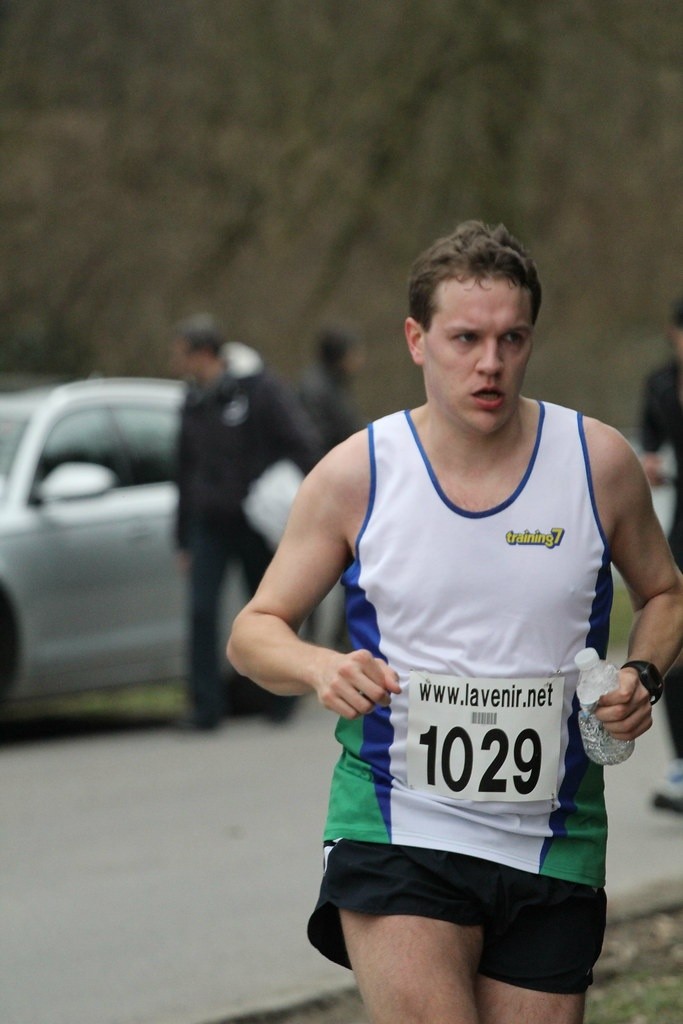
[0,379,346,700]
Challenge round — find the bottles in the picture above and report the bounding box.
[574,647,634,764]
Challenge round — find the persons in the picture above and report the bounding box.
[640,296,683,814]
[226,218,683,1024]
[172,323,367,735]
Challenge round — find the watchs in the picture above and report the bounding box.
[620,660,664,706]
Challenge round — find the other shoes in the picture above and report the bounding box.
[652,759,683,808]
